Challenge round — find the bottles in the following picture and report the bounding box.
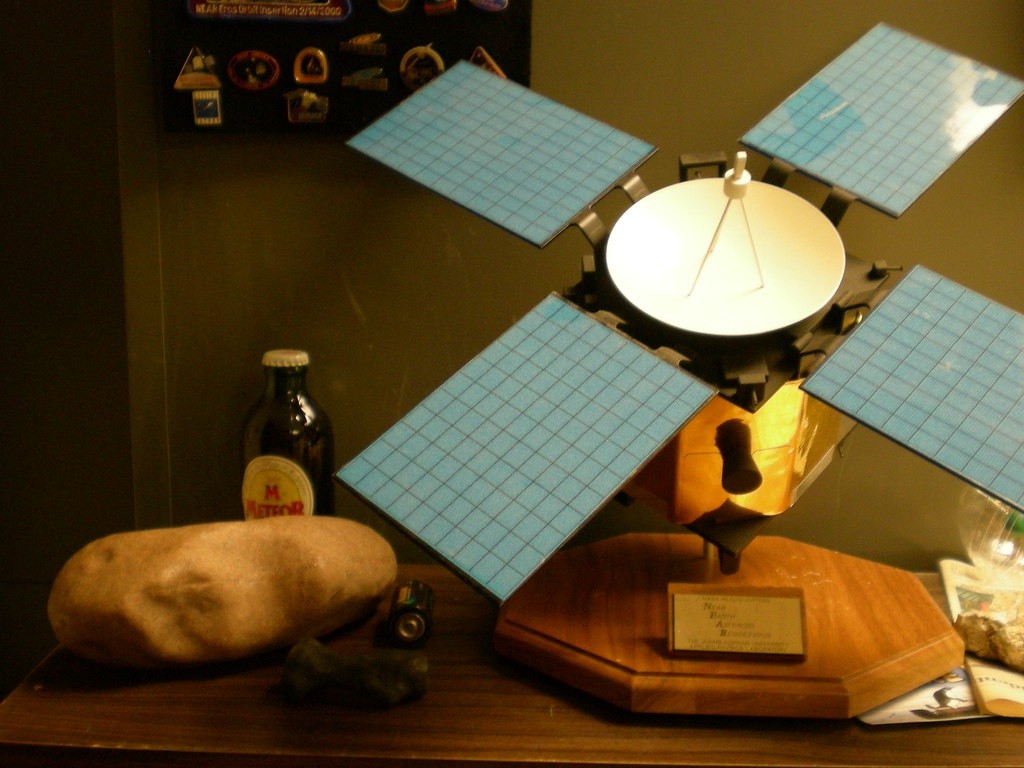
[238,348,335,521]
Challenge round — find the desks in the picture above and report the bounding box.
[2,562,1023,767]
